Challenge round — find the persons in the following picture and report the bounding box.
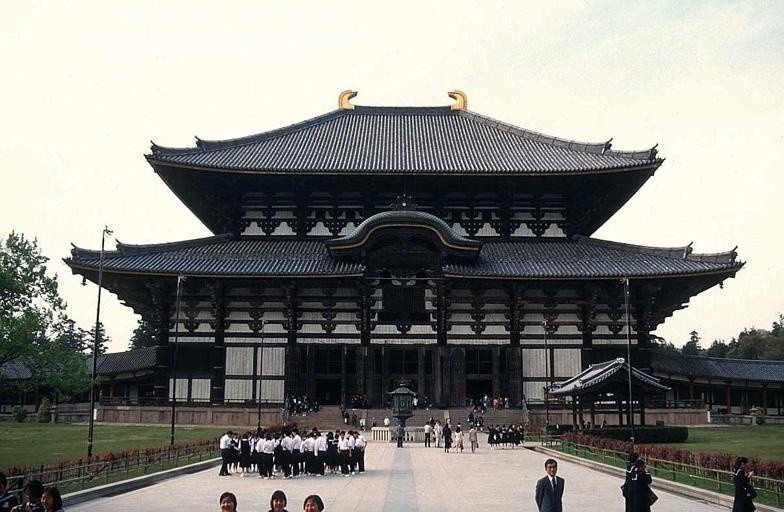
[303,494,325,511]
[0,472,18,512]
[339,392,396,431]
[40,487,64,511]
[731,456,758,511]
[267,490,289,512]
[219,426,369,480]
[624,452,639,480]
[623,459,653,511]
[482,393,511,413]
[412,394,484,453]
[11,480,45,511]
[219,492,237,512]
[534,459,566,511]
[488,423,524,450]
[280,392,322,417]
